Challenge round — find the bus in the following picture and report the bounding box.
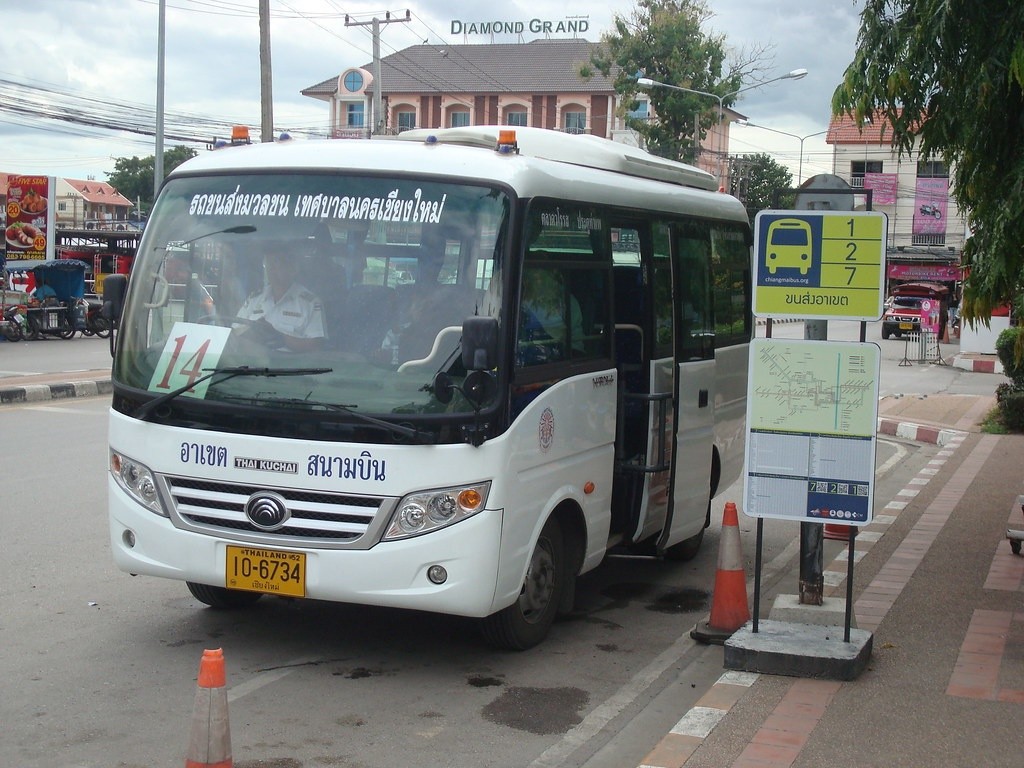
[107,122,756,653]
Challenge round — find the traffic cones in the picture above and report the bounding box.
[688,502,750,644]
[823,524,860,541]
[185,647,234,768]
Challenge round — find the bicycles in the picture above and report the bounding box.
[0,305,39,341]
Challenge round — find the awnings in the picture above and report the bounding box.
[6,258,92,306]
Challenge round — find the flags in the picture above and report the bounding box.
[887,264,967,282]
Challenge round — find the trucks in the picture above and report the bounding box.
[880,279,951,341]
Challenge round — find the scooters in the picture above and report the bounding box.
[920,201,941,219]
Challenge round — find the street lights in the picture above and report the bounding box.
[735,117,872,187]
[636,69,808,183]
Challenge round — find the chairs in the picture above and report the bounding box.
[40,296,61,307]
[312,253,708,357]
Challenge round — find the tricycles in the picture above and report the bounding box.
[82,303,116,337]
[4,257,90,337]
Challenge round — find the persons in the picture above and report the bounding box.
[478,236,585,362]
[884,294,894,307]
[948,291,960,324]
[33,278,55,306]
[232,239,331,350]
[379,292,435,362]
